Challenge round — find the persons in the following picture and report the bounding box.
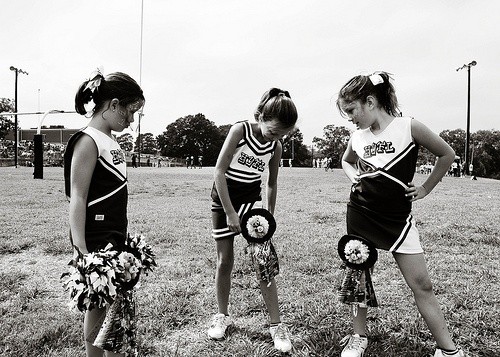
[337,70,468,357]
[64,70,146,357]
[312,157,333,173]
[131,152,203,169]
[420,156,474,178]
[207,88,298,353]
[0,138,67,168]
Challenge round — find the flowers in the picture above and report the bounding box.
[245,214,270,239]
[343,239,370,264]
[127,232,159,277]
[60,242,126,313]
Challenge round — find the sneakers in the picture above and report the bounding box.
[434,349,470,357]
[133,166,204,169]
[420,175,472,178]
[341,334,368,357]
[270,325,292,352]
[210,313,236,341]
[281,168,334,173]
